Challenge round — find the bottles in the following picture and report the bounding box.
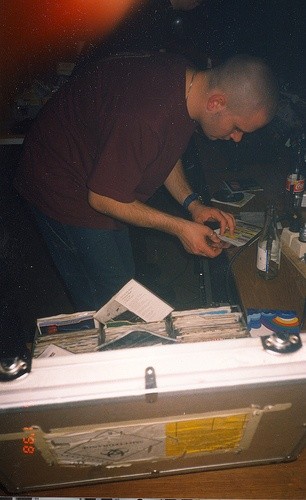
[299,223,306,242]
[256,203,281,280]
[284,168,305,212]
[289,215,300,232]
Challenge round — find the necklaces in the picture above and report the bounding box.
[186,71,195,102]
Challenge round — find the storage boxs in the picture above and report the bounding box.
[0,303,306,494]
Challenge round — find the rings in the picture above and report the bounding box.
[209,229,214,238]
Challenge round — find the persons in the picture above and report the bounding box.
[14,50,278,312]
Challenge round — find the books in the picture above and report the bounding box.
[214,217,265,247]
[32,279,251,363]
[211,176,264,208]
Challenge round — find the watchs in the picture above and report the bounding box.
[183,191,203,210]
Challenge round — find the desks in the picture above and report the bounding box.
[196,126,306,332]
